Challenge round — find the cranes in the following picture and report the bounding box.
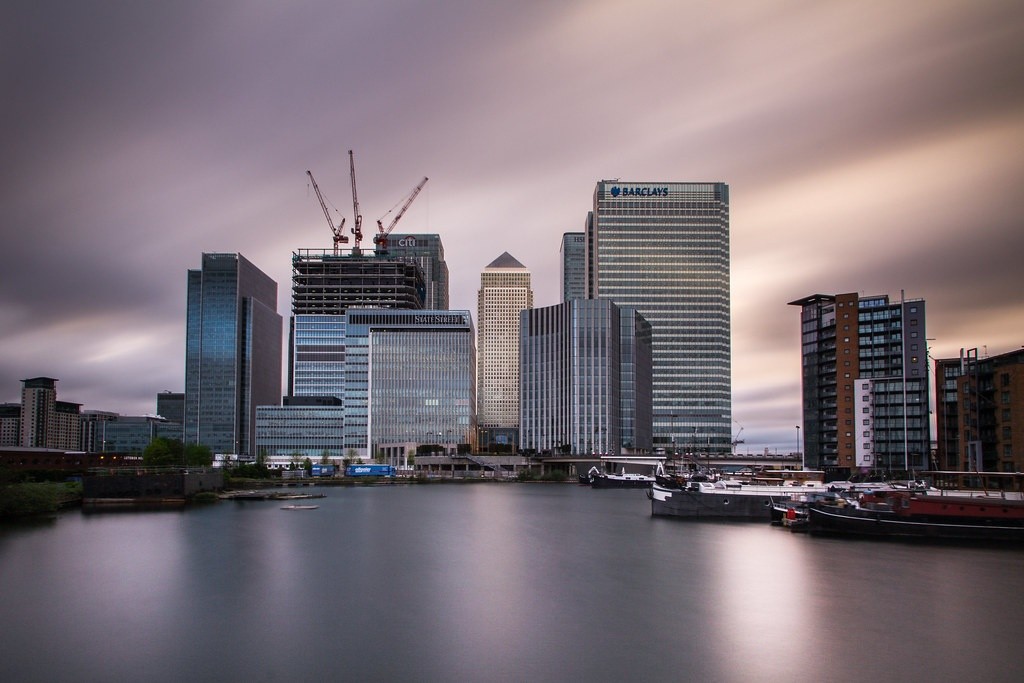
[306,169,349,256]
[372,176,429,253]
[347,149,363,256]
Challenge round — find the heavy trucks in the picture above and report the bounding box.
[311,464,334,476]
[346,463,396,478]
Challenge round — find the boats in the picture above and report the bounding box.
[645,465,825,518]
[280,504,319,511]
[805,477,1024,543]
[590,467,656,488]
[772,492,842,517]
[273,490,327,499]
